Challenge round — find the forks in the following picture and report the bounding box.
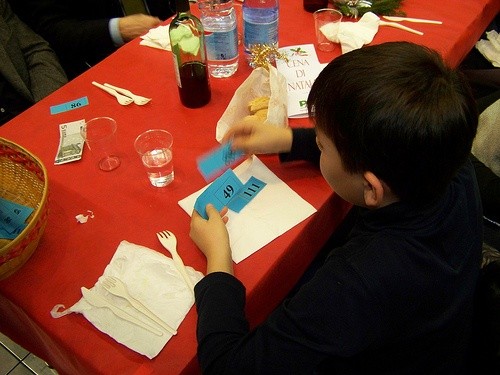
[100,276,177,335]
[156,230,194,292]
[92,81,134,105]
[104,83,152,105]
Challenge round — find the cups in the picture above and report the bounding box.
[134,129,175,187]
[314,8,342,51]
[81,117,120,170]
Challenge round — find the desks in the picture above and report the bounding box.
[0,0,500,375]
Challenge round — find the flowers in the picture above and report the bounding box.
[330,0,406,19]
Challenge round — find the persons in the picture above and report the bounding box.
[190,41,500,375]
[0,0,190,125]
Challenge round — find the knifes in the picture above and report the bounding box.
[81,287,164,336]
[383,16,442,24]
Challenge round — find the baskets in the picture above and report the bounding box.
[0,137,48,281]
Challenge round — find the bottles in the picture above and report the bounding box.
[199,0,239,78]
[304,0,328,12]
[169,0,210,108]
[242,0,280,67]
